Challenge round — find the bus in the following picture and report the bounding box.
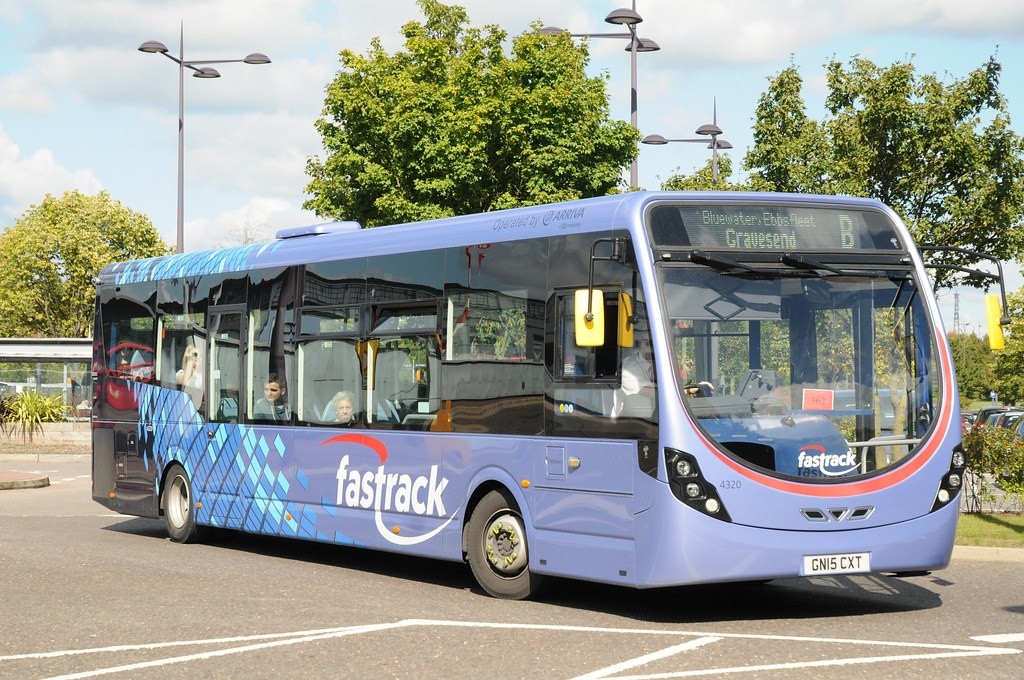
[93,201,973,602]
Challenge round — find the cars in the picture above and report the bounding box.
[960,406,1024,440]
[828,389,940,456]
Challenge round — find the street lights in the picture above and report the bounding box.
[139,18,272,255]
[642,95,730,193]
[536,1,661,193]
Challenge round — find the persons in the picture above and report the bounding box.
[328,390,356,427]
[173,344,206,415]
[609,324,715,417]
[252,373,293,424]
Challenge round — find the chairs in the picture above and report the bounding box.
[128,347,400,424]
